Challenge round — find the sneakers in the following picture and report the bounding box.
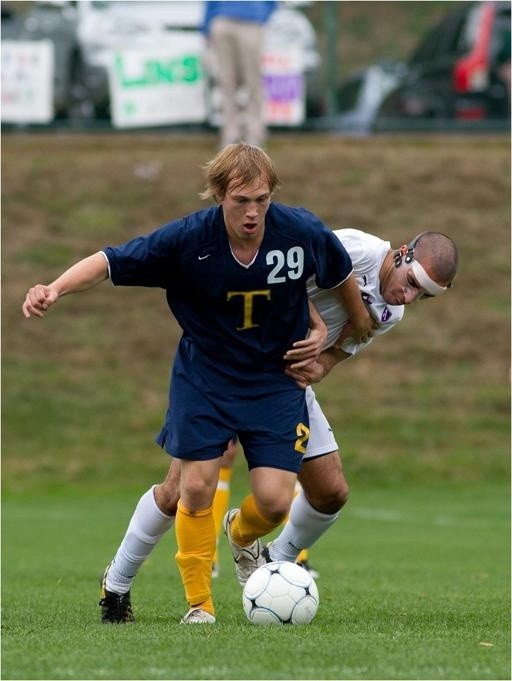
[98,562,136,626]
[178,603,216,627]
[211,507,322,591]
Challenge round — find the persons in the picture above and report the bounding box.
[96,227,464,628]
[21,140,377,634]
[200,1,278,153]
[211,435,326,583]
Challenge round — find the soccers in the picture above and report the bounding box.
[242,562,319,627]
